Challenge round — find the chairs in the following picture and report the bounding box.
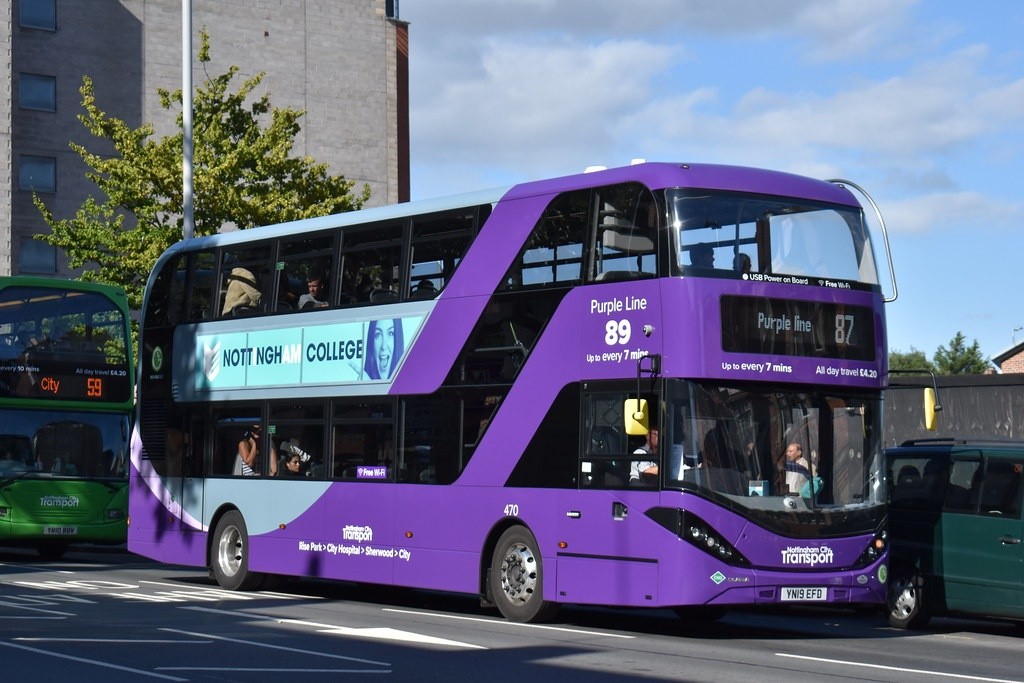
[342,467,444,480]
[200,285,439,319]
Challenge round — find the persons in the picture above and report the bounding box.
[221,247,435,318]
[689,243,754,271]
[628,417,822,501]
[231,420,442,478]
[13,331,48,365]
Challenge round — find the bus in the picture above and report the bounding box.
[0,274,142,561]
[127,159,943,628]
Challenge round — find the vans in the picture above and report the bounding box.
[886,436,1024,633]
